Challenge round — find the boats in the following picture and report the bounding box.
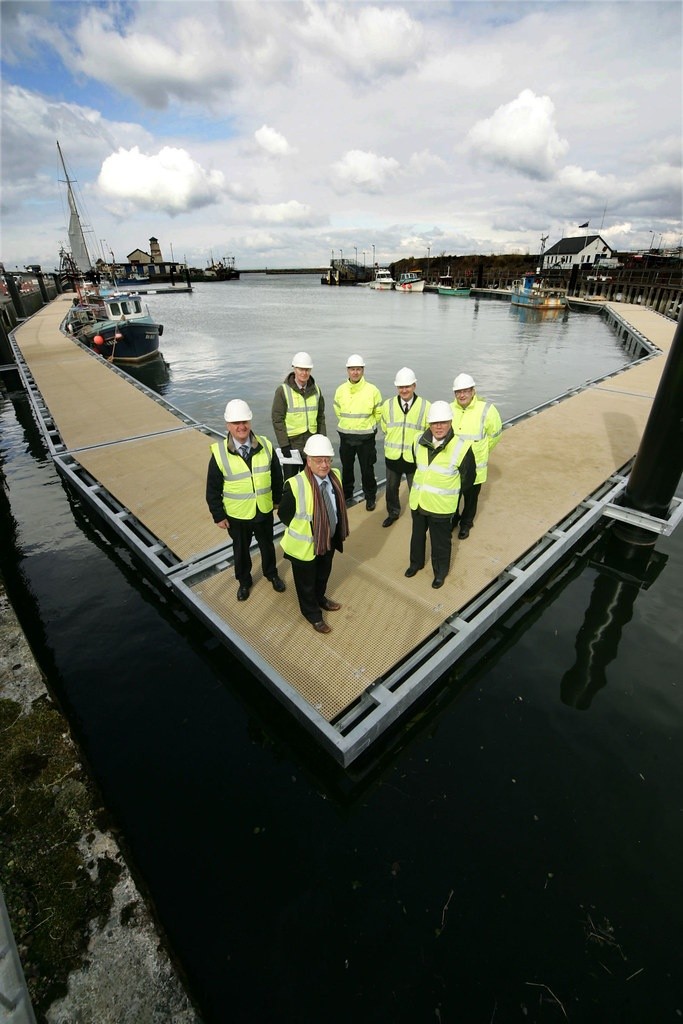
[439,287,471,296]
[357,269,394,290]
[80,266,150,290]
[220,258,240,281]
[395,273,425,291]
[321,258,372,286]
[511,271,569,310]
[424,276,453,292]
[66,291,170,387]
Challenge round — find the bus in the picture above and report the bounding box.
[409,270,423,277]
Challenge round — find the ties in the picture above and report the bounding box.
[321,481,336,537]
[240,445,249,461]
[405,402,408,412]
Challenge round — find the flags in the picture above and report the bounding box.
[579,221,589,228]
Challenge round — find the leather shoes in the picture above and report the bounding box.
[366,499,375,511]
[458,526,469,540]
[322,599,340,611]
[238,581,253,600]
[268,575,285,592]
[313,620,331,634]
[431,577,444,588]
[383,516,399,528]
[405,566,424,577]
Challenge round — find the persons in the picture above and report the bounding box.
[333,354,382,511]
[206,399,286,601]
[382,367,432,527]
[277,434,350,633]
[405,400,476,589]
[449,373,502,540]
[271,352,327,483]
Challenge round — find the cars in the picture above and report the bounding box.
[4,276,24,286]
[203,268,217,277]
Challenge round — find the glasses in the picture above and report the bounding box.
[311,457,334,464]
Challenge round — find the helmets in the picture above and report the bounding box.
[452,373,476,391]
[427,401,453,424]
[394,367,417,387]
[346,354,365,367]
[225,399,253,421]
[303,434,334,456]
[292,352,314,369]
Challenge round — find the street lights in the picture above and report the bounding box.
[100,240,108,263]
[354,248,357,266]
[363,253,365,268]
[372,244,375,267]
[427,248,431,276]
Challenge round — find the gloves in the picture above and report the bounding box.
[281,445,293,458]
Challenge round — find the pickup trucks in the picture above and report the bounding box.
[20,279,50,293]
